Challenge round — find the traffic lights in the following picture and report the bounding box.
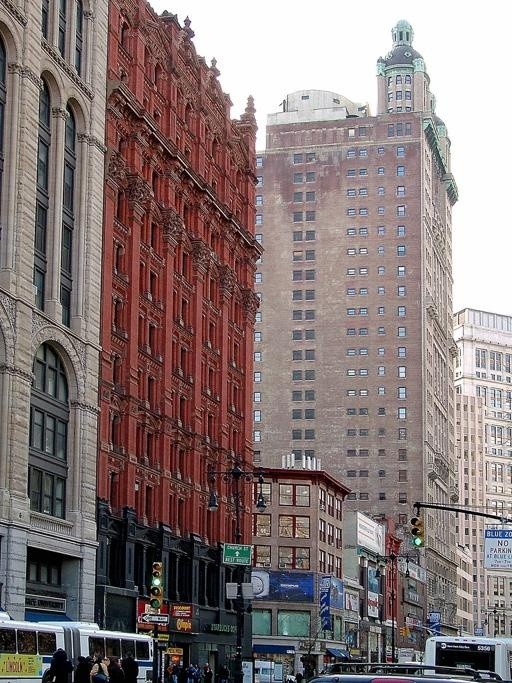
[406,626,410,637]
[410,516,425,548]
[399,627,405,636]
[149,585,164,609]
[151,561,163,585]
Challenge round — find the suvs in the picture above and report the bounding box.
[307,661,510,681]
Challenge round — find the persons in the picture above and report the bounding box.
[74,651,138,683]
[50,648,73,683]
[204,663,212,683]
[187,664,201,683]
[295,671,302,683]
[165,664,179,683]
[221,665,230,683]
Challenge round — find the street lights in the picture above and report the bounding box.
[372,548,420,662]
[482,612,492,635]
[207,460,269,682]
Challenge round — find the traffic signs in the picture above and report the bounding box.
[141,613,170,623]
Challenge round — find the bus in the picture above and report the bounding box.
[423,636,511,679]
[1,611,157,682]
